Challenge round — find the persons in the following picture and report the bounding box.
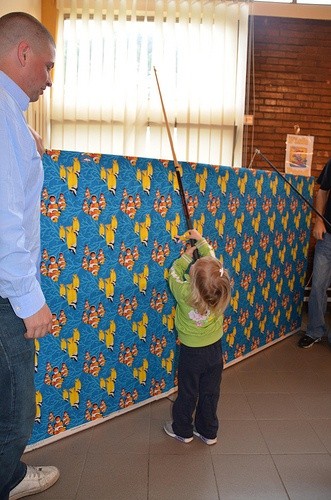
[164,230,233,446]
[0,10,70,499]
[296,155,331,352]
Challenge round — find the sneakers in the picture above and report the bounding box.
[7,462,60,500]
[299,334,323,350]
[189,425,218,446]
[164,421,194,444]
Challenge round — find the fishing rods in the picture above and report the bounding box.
[153,65,201,263]
[255,150,331,228]
